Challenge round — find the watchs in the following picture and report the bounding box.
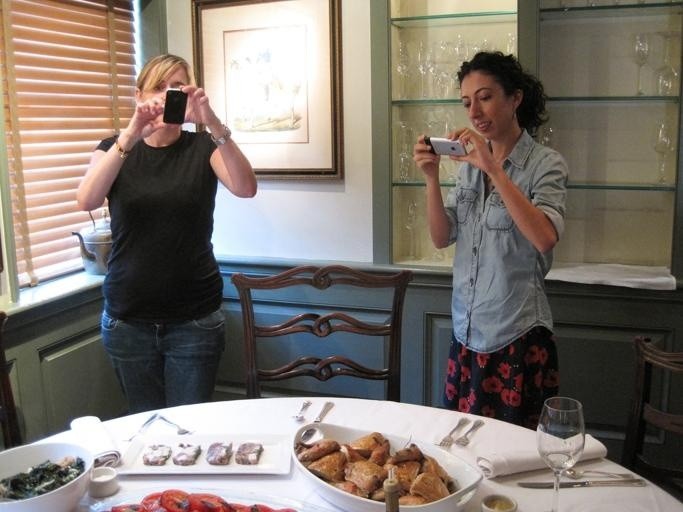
[210,124,231,146]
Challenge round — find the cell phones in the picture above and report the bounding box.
[163,90,187,125]
[423,135,468,158]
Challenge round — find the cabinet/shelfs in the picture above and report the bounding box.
[387,0,516,267]
[537,1,683,290]
[0,284,127,446]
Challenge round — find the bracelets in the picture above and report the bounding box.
[115,138,131,160]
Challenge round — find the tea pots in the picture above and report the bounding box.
[73,224,112,274]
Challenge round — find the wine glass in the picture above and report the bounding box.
[654,30,678,96]
[393,107,463,181]
[654,121,673,186]
[539,127,556,150]
[397,189,452,261]
[394,33,516,98]
[632,33,650,94]
[536,395,585,508]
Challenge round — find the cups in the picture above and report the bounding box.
[90,465,118,498]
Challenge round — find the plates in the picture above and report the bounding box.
[116,432,294,475]
[293,423,482,511]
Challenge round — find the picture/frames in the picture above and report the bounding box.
[192,0,345,179]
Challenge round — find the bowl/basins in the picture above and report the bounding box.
[0,443,94,512]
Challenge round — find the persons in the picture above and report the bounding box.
[74,53,257,415]
[413,50,570,431]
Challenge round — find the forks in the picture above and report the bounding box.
[434,417,468,447]
[157,416,187,434]
[455,419,483,447]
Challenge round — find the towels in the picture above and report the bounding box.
[69,415,122,470]
[475,432,609,479]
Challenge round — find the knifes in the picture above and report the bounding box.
[515,480,646,489]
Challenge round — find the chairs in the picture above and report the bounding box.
[231,265,414,403]
[620,335,682,501]
[0,312,23,448]
